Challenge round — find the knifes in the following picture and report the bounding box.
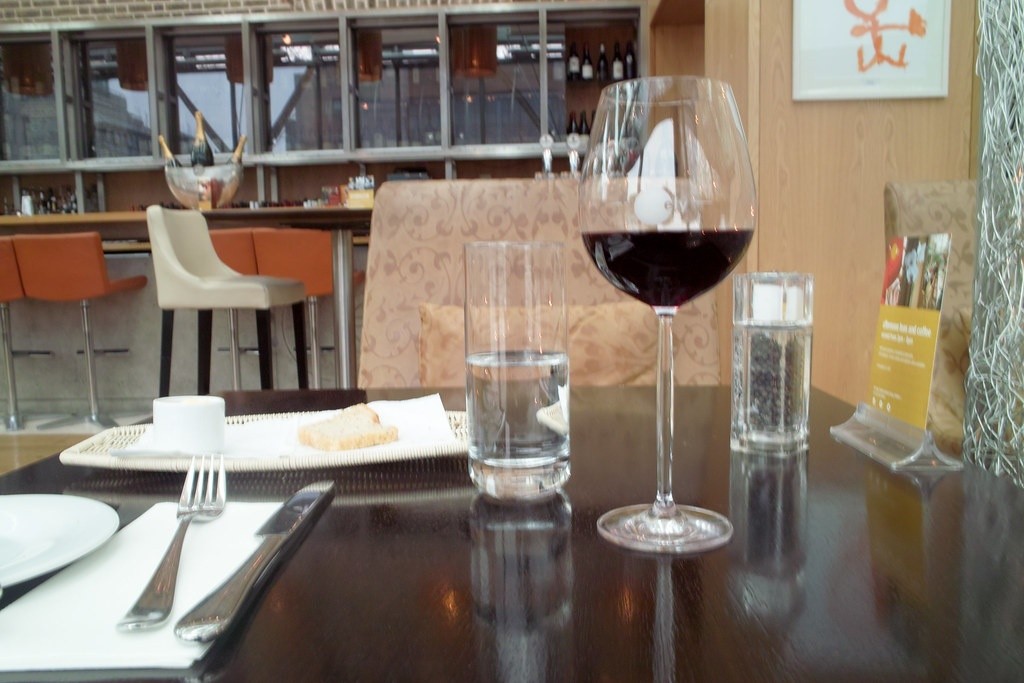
[174,479,334,643]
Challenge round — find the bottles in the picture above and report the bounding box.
[578,111,589,135]
[580,40,593,81]
[226,135,247,166]
[21,186,76,216]
[567,112,578,134]
[568,41,580,80]
[191,111,215,167]
[611,40,624,81]
[624,40,635,79]
[597,42,608,81]
[157,134,181,167]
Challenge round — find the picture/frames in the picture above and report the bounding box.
[791,0,952,102]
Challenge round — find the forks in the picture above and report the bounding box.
[115,455,226,629]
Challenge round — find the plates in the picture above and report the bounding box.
[0,493,120,589]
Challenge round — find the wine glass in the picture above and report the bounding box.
[577,74,756,553]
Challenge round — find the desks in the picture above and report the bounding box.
[2,384,1024,683]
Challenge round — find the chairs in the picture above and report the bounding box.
[357,175,723,386]
[884,178,976,456]
[147,203,363,400]
[0,232,145,430]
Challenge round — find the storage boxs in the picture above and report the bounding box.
[341,184,374,209]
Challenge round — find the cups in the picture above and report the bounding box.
[463,241,572,504]
[730,272,814,458]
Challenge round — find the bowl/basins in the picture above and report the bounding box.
[164,167,244,209]
[150,395,226,454]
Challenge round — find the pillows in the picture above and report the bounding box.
[416,301,662,386]
